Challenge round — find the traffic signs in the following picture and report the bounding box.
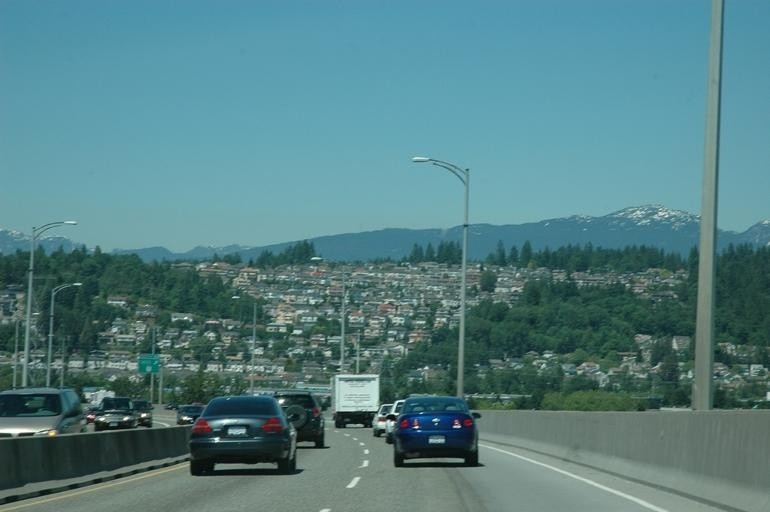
[138,354,159,374]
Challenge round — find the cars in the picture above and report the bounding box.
[177,405,205,425]
[371,397,480,467]
[0,386,153,438]
[188,396,297,477]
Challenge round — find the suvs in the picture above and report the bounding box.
[273,389,324,448]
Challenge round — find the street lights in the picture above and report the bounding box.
[22,221,77,387]
[46,282,82,387]
[232,295,257,395]
[412,156,471,396]
[311,257,346,375]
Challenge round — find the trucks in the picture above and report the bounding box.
[330,374,380,428]
